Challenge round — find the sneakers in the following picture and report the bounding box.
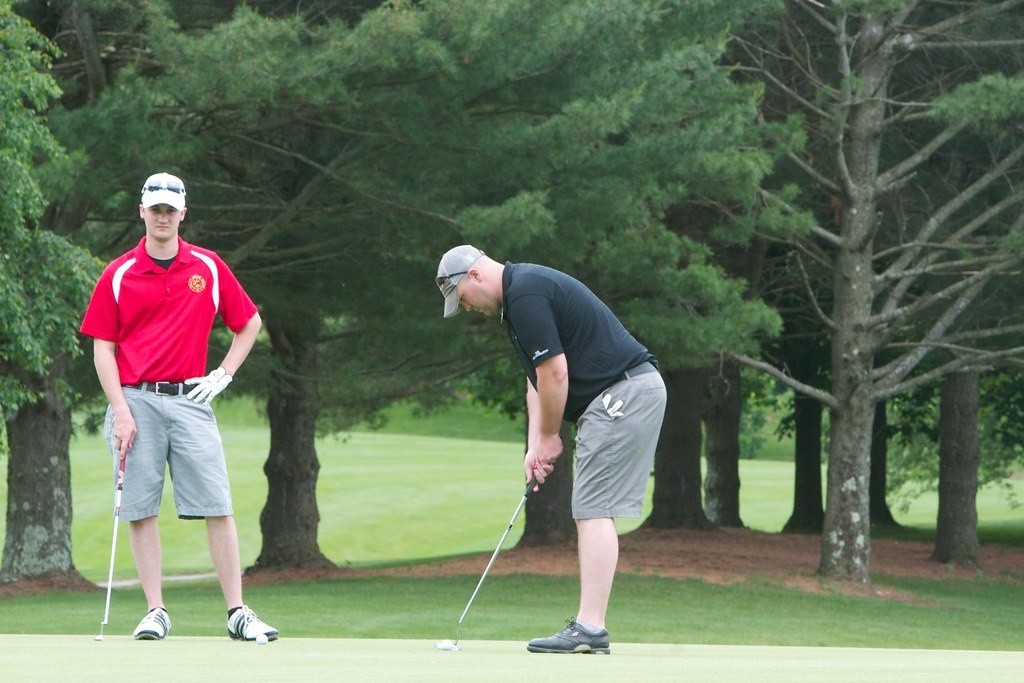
[226,605,279,641]
[132,608,172,640]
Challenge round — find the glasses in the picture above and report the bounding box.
[143,180,185,194]
[435,271,468,288]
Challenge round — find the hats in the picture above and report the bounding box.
[141,172,186,212]
[434,245,486,318]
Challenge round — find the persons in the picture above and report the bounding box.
[79,173,280,641]
[435,245,668,655]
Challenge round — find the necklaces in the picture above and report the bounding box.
[501,307,503,325]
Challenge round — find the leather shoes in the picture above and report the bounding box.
[526,616,610,655]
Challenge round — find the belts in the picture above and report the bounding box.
[594,361,658,391]
[121,379,202,398]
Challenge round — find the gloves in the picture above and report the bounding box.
[183,367,233,406]
[603,394,625,420]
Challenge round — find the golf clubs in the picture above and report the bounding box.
[434,473,539,651]
[94,446,128,643]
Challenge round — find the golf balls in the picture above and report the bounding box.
[441,639,453,651]
